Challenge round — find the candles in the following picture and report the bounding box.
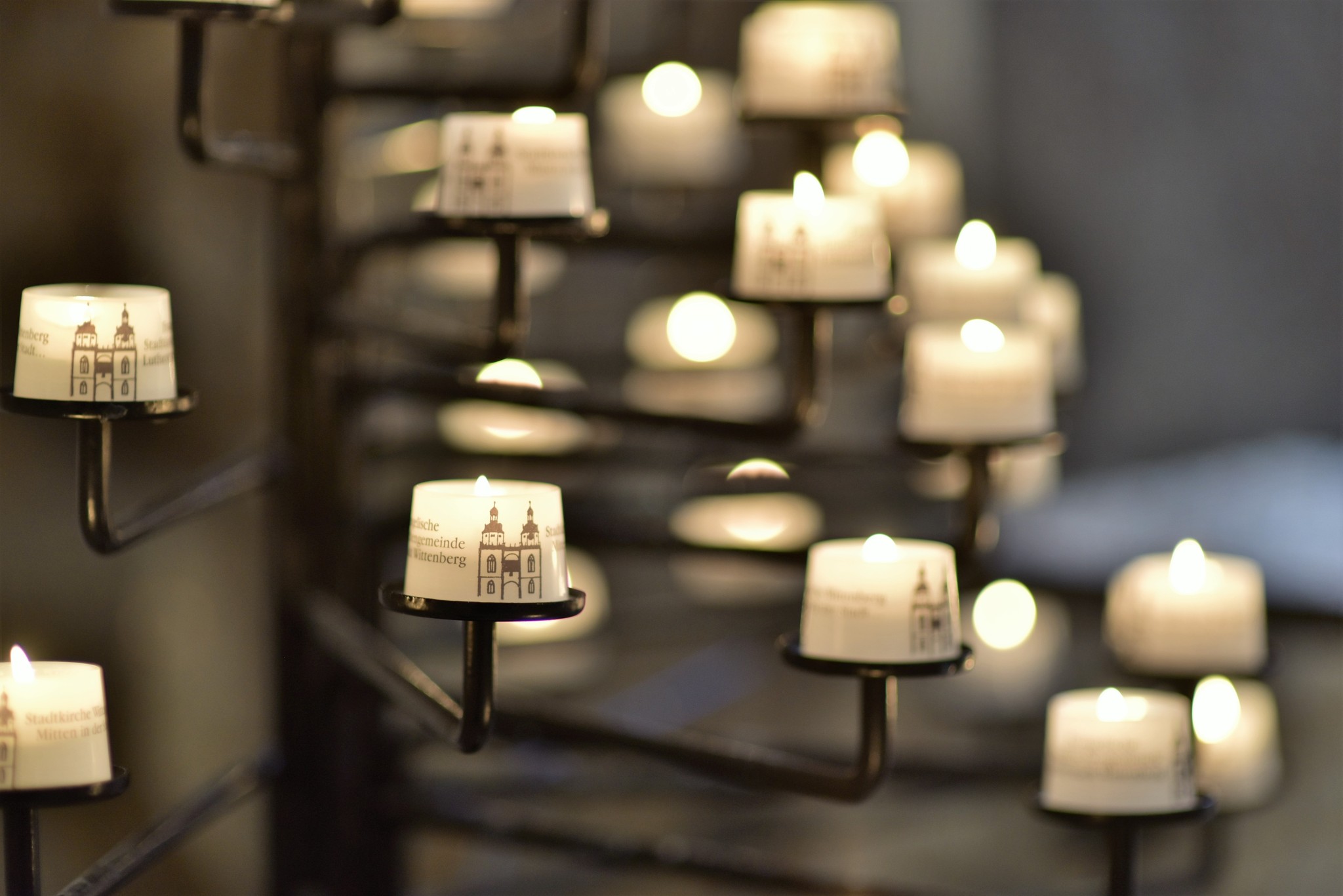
[401,1,1284,814]
[0,646,118,792]
[13,285,178,400]
